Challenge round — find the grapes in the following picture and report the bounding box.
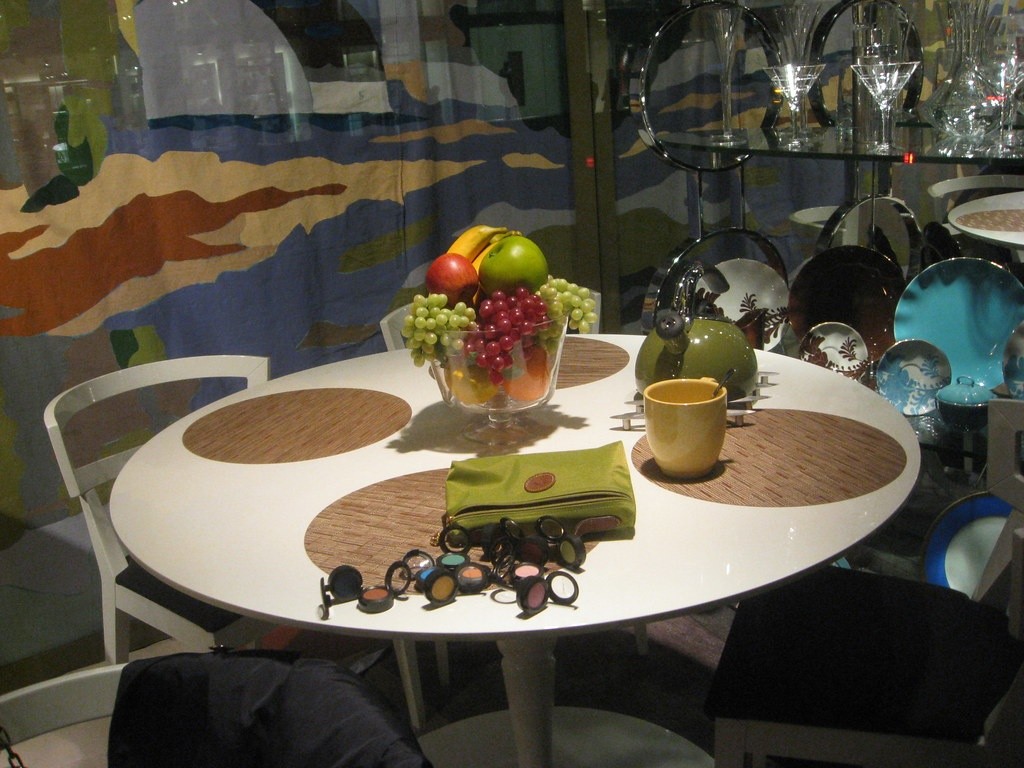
[400,278,599,387]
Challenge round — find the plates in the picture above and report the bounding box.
[694,174,1024,417]
[920,488,1011,600]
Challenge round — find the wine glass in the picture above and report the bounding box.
[704,0,1024,160]
[430,310,570,447]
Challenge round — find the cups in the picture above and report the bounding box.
[642,376,728,480]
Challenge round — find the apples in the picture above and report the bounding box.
[478,235,549,296]
[442,361,499,404]
[425,253,479,305]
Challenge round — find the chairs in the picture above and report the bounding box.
[110,333,924,768]
[41,353,278,663]
[705,398,1024,768]
[380,283,650,692]
[0,653,434,768]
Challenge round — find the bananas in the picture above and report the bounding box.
[445,224,523,303]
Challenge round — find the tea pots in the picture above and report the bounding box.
[634,297,758,408]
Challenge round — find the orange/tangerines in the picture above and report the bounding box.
[498,348,550,400]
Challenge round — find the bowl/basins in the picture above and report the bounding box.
[936,376,997,434]
